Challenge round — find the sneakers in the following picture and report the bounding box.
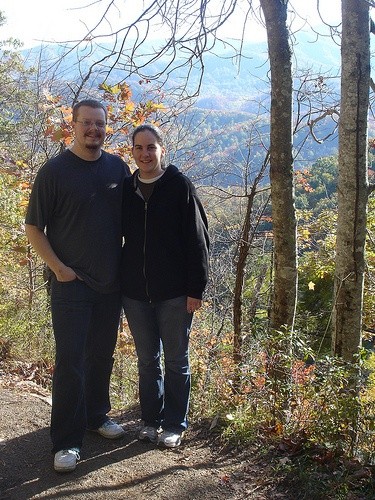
[163,431,185,447]
[54,448,80,473]
[86,419,125,439]
[139,426,163,442]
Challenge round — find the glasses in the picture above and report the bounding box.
[77,120,107,128]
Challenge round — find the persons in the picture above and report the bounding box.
[119,124,208,449]
[24,99,130,472]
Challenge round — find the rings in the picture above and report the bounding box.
[196,305,199,308]
